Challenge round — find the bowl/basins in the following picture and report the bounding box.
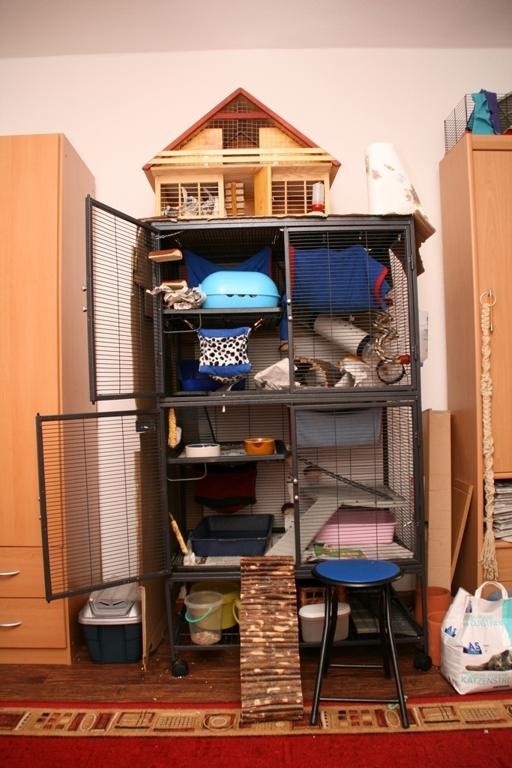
[186,443,220,457]
[244,437,276,456]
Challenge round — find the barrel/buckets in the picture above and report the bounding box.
[231,596,240,624]
[185,591,225,645]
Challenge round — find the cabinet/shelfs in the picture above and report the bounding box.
[0,131,512,667]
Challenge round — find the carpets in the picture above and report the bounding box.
[0,697,512,768]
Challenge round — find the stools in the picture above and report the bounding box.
[308,558,409,728]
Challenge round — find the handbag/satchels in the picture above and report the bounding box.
[439,578,511,697]
